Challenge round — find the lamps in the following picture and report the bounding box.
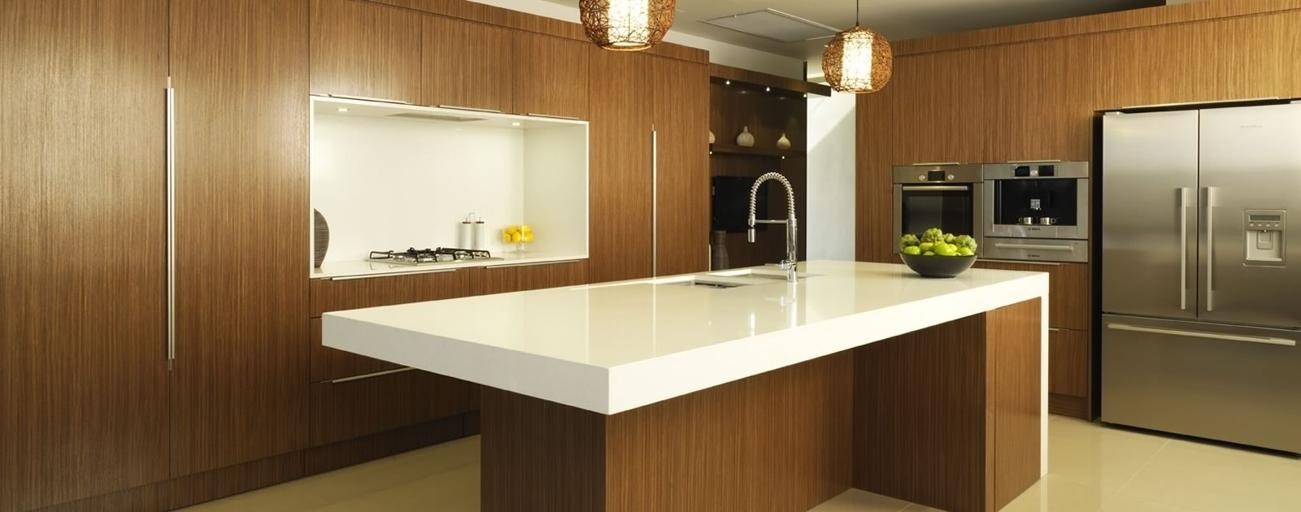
[819,0,893,96]
[579,1,676,51]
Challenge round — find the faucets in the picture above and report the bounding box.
[768,282,799,326]
[746,171,800,282]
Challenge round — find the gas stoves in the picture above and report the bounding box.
[368,245,505,269]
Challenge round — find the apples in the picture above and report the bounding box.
[901,227,978,256]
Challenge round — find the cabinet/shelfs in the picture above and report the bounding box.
[588,38,712,279]
[984,260,1096,422]
[1,0,310,508]
[310,311,482,479]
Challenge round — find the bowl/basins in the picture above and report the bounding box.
[898,249,980,279]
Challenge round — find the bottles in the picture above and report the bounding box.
[459,216,473,251]
[474,218,485,251]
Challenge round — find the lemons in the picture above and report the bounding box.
[502,225,534,243]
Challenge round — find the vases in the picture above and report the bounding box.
[775,132,792,150]
[737,125,755,147]
[707,127,714,144]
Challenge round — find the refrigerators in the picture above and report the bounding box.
[1101,99,1301,457]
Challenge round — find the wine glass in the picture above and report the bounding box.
[499,225,537,256]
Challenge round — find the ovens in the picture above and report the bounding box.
[890,162,982,261]
[983,164,1089,263]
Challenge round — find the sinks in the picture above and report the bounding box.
[730,268,807,282]
[666,277,746,291]
